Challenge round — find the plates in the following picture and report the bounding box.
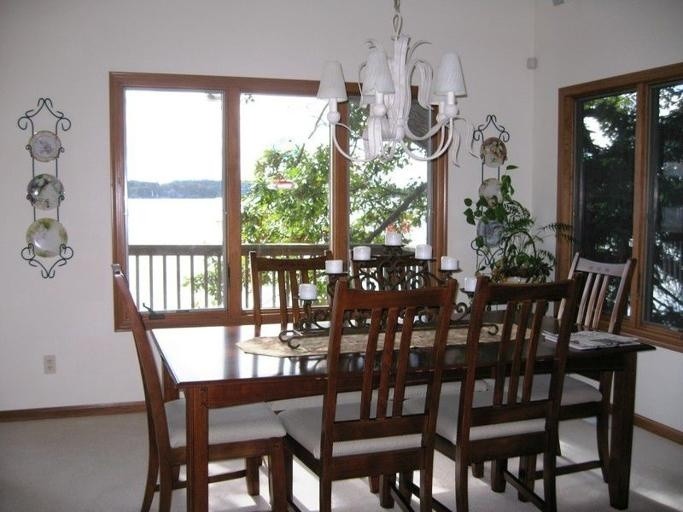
[29,130,61,162]
[24,218,68,257]
[478,177,506,208]
[25,174,64,210]
[479,137,507,168]
[475,217,505,249]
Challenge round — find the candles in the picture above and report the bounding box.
[440,255,460,270]
[464,277,478,292]
[352,246,370,261]
[323,259,343,274]
[415,244,432,261]
[384,232,402,246]
[298,285,317,299]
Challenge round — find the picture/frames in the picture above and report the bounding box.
[312,0,477,169]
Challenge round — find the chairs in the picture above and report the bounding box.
[390,274,577,510]
[245,249,362,412]
[108,263,289,509]
[351,255,487,401]
[275,275,460,512]
[488,249,639,504]
